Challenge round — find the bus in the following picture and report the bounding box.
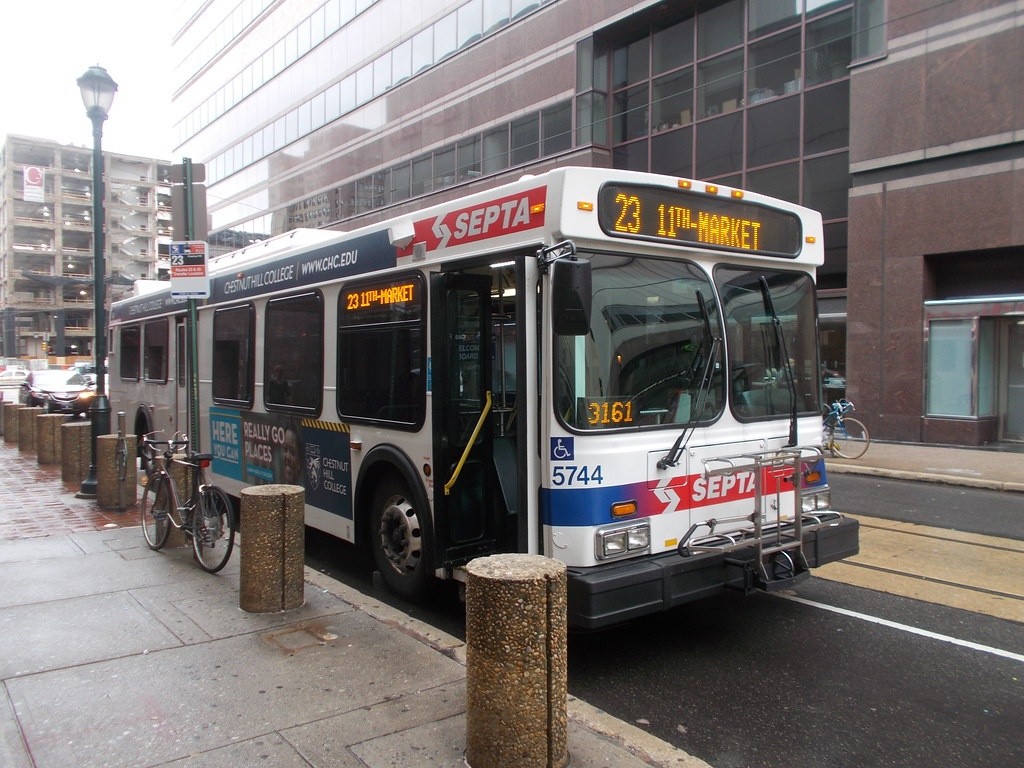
[108,165,861,635]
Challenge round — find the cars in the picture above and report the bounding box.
[0,365,30,387]
[18,368,96,419]
[70,359,109,400]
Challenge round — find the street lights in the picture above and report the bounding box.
[76,63,120,500]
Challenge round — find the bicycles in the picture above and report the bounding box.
[137,429,237,574]
[820,397,870,459]
